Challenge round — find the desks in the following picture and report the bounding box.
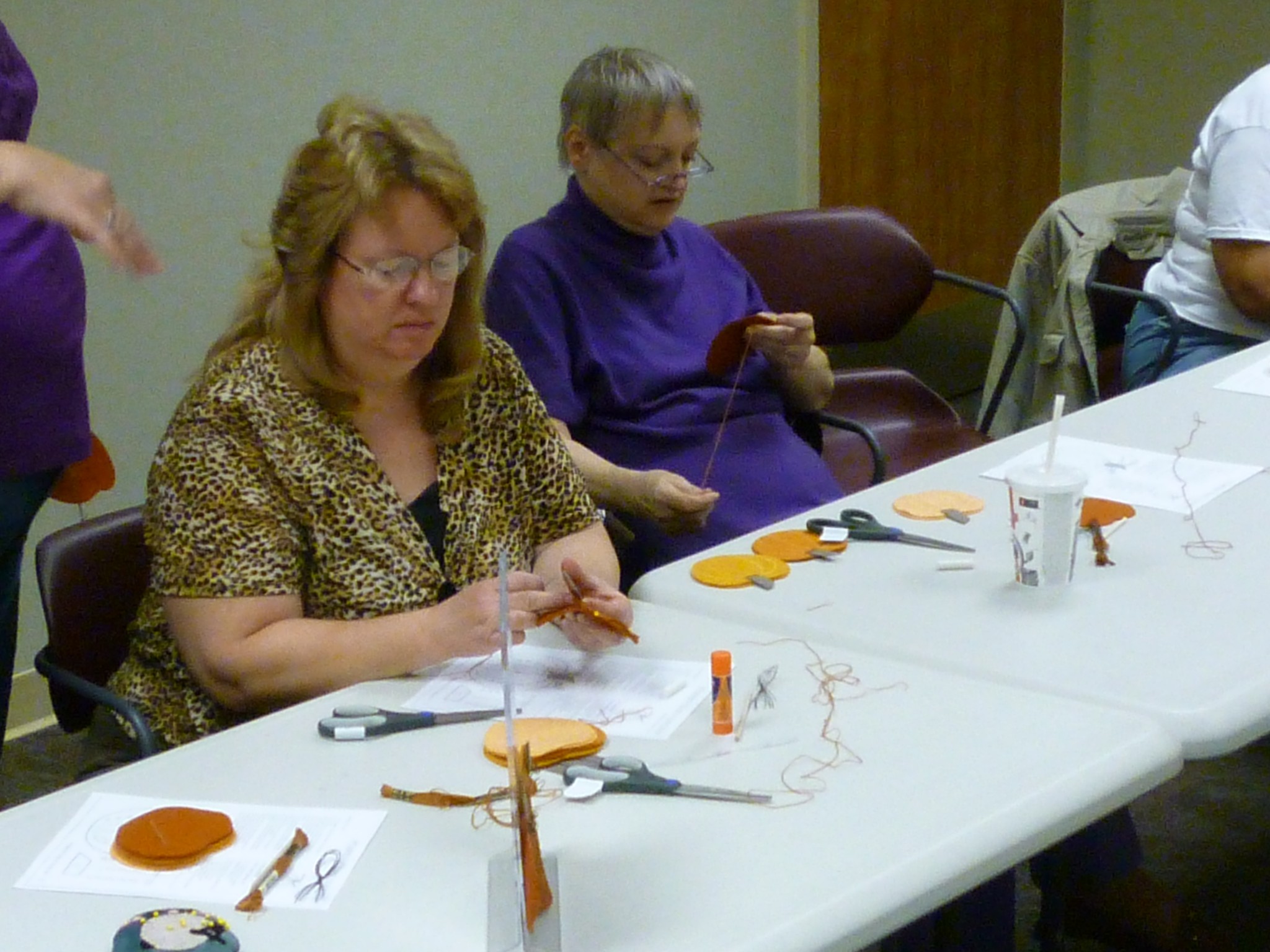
[633,332,1270,757]
[0,592,1180,952]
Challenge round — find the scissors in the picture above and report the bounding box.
[808,510,976,552]
[318,701,523,743]
[563,756,771,804]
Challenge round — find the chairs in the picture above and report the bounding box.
[34,503,173,756]
[975,167,1195,441]
[701,207,1026,496]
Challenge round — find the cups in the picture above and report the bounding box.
[1003,462,1090,588]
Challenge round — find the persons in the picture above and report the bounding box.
[1121,59,1270,395]
[1,18,161,746]
[483,47,1210,951]
[74,100,633,783]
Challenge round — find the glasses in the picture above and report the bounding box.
[596,134,713,189]
[325,245,473,289]
